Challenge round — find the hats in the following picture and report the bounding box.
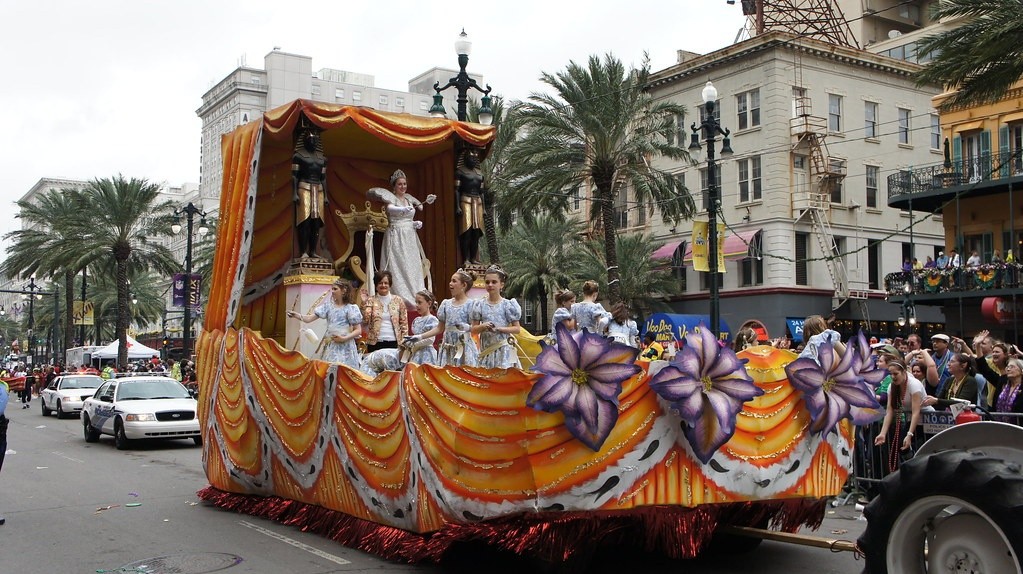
[939,252,943,256]
[877,344,899,356]
[931,334,950,343]
[644,331,656,339]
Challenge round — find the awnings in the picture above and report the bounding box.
[787,318,806,342]
[639,313,733,342]
[649,229,761,271]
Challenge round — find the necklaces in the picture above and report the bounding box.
[889,372,908,475]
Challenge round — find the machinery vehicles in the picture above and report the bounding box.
[853,402,1022,574]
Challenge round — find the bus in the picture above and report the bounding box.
[65,346,109,371]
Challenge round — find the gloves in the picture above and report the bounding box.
[455,322,469,332]
[402,335,422,348]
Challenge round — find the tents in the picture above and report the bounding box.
[91,332,161,371]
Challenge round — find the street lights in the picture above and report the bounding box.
[687,77,736,339]
[126,282,139,334]
[166,200,210,379]
[426,26,497,126]
[20,279,44,370]
[893,276,917,339]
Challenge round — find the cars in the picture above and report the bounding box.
[78,373,203,451]
[40,371,104,419]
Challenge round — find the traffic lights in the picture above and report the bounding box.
[168,337,175,349]
[161,335,168,348]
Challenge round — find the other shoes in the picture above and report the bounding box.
[858,493,869,503]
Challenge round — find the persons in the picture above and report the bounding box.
[867,331,1023,427]
[410,268,480,368]
[285,277,364,372]
[0,351,200,409]
[362,269,409,373]
[798,315,842,367]
[874,360,935,450]
[0,379,9,525]
[471,262,523,372]
[571,279,602,336]
[361,340,416,377]
[642,331,664,361]
[406,290,439,370]
[378,169,423,310]
[901,248,1020,295]
[551,288,577,342]
[732,327,761,354]
[608,303,639,349]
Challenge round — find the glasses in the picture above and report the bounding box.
[1005,365,1014,371]
[906,342,915,346]
